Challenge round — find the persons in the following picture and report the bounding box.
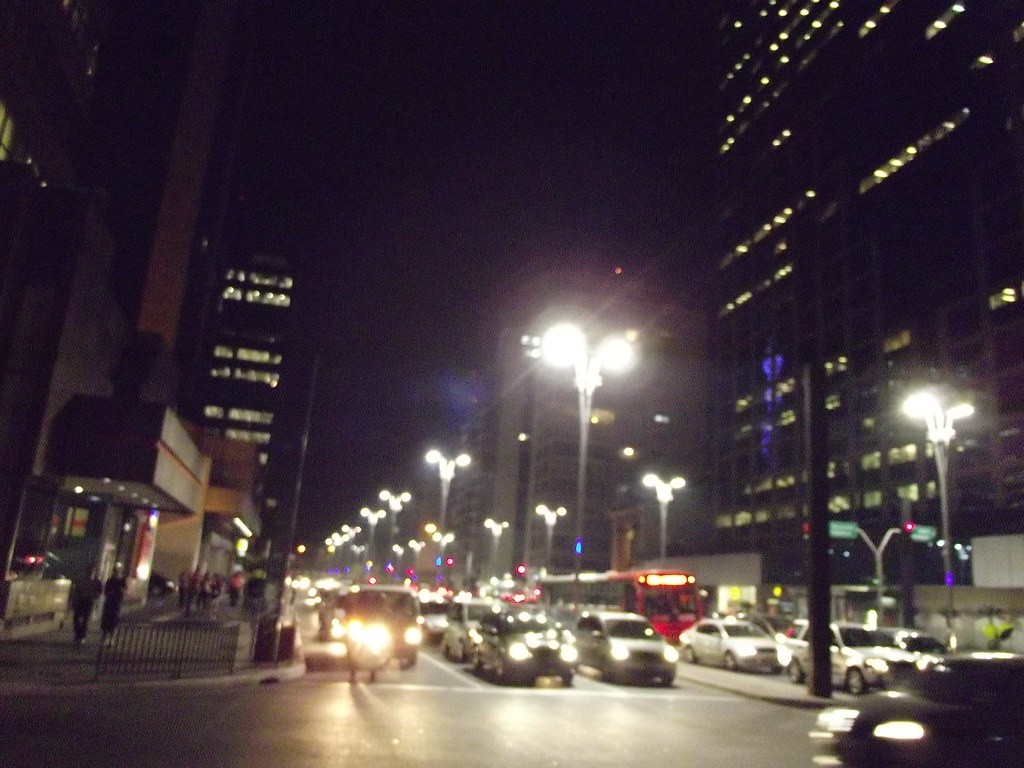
[179,569,242,622]
[100,567,130,645]
[72,565,103,647]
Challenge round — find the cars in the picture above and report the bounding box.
[808,653,1024,768]
[473,613,578,684]
[577,610,679,686]
[678,620,782,672]
[319,576,679,685]
[740,612,947,695]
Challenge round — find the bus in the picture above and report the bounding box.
[536,571,702,642]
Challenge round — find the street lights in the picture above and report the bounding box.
[536,506,566,571]
[905,393,975,617]
[542,326,636,609]
[483,518,509,574]
[641,474,686,569]
[360,507,386,567]
[426,450,472,570]
[380,490,412,561]
